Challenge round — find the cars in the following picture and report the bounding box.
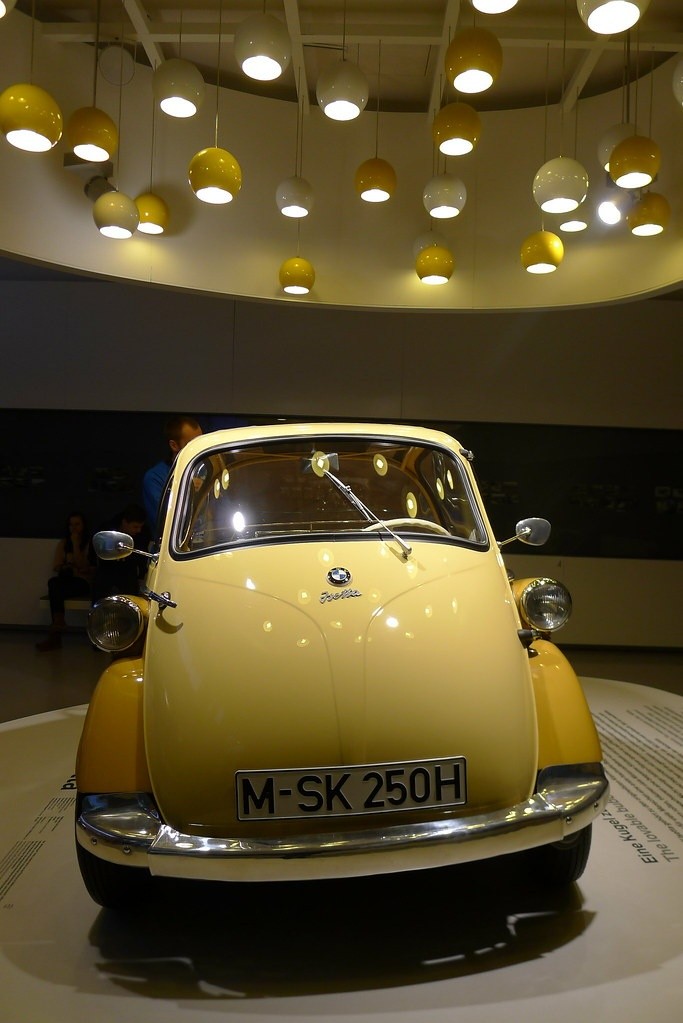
[76,422,608,909]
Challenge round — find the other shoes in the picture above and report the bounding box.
[36,636,62,652]
[48,613,67,637]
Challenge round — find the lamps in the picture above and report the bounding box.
[1,0,669,298]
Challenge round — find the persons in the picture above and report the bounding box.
[34,513,100,649]
[141,415,203,534]
[89,506,149,609]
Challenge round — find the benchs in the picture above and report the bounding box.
[41,593,148,601]
